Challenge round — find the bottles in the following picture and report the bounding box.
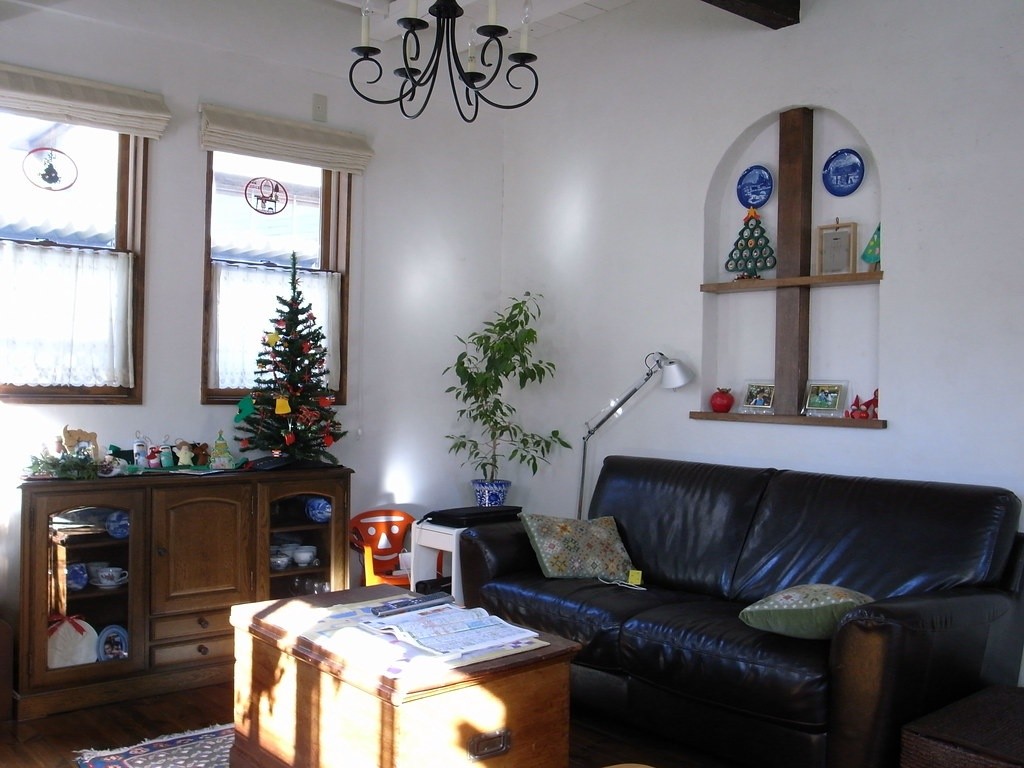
[711,386,734,413]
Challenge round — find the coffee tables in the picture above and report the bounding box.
[229,583,583,768]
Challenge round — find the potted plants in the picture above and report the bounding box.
[442,291,574,507]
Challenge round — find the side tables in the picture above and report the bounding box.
[410,521,466,608]
[901,684,1024,768]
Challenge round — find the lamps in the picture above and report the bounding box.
[348,0,538,123]
[576,352,696,520]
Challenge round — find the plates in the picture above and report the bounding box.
[66,563,89,592]
[305,497,332,523]
[89,578,128,588]
[737,165,773,209]
[821,149,865,197]
[105,510,129,538]
[97,624,129,661]
[47,619,99,669]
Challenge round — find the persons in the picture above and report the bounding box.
[750,388,766,405]
[817,388,833,406]
[105,640,121,657]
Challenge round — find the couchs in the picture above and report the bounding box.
[459,456,1024,768]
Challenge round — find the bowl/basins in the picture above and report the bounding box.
[269,543,317,570]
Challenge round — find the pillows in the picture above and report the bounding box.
[516,512,643,583]
[739,582,876,639]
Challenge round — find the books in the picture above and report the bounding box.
[359,602,538,659]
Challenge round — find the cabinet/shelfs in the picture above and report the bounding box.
[0,466,355,722]
[689,108,888,430]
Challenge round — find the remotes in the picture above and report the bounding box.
[372,592,455,617]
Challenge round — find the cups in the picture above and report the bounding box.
[86,562,129,583]
[286,576,330,596]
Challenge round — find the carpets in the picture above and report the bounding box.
[71,723,235,768]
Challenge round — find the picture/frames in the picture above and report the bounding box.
[801,380,849,414]
[738,379,775,415]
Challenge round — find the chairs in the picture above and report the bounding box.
[349,510,443,586]
